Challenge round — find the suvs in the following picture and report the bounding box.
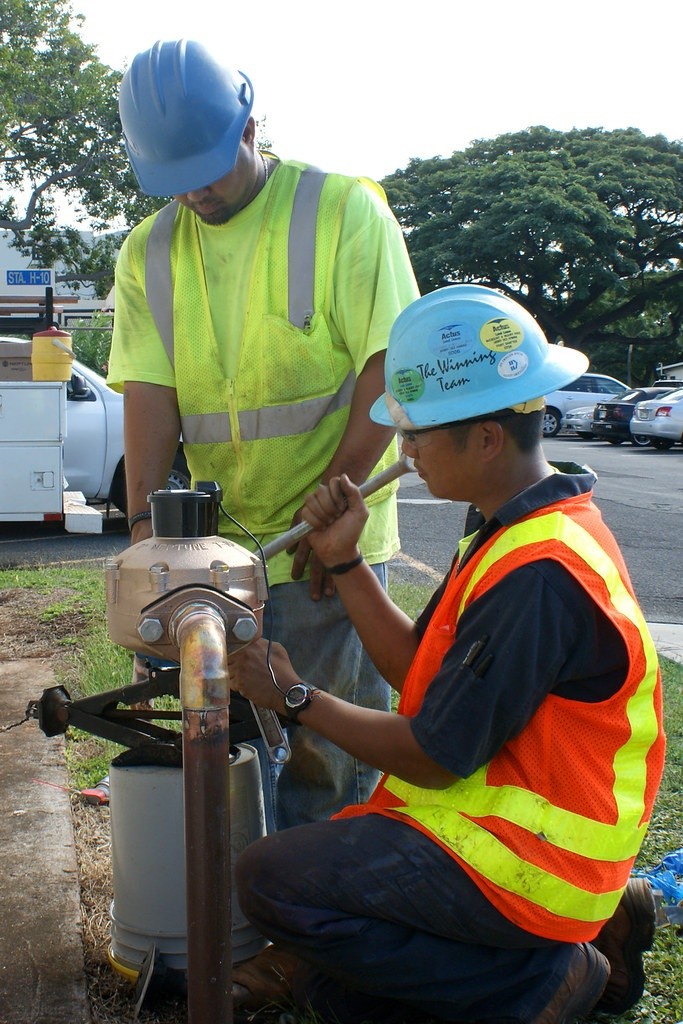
[543,373,632,439]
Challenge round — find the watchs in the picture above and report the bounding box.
[282,682,317,727]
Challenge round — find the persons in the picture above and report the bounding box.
[107,38,422,833]
[232,284,666,1024]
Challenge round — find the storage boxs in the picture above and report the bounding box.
[0,341,33,381]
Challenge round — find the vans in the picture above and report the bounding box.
[0,336,191,516]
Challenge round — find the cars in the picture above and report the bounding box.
[561,404,597,440]
[589,379,683,448]
[629,387,683,452]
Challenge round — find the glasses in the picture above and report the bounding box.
[397,411,501,449]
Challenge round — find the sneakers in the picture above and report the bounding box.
[531,940,610,1024]
[589,878,657,1015]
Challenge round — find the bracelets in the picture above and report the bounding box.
[328,552,363,576]
[128,511,152,531]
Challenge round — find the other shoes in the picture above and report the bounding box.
[232,945,312,1004]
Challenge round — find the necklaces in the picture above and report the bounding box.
[257,150,269,181]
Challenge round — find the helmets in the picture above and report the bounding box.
[369,284,590,427]
[119,38,254,195]
